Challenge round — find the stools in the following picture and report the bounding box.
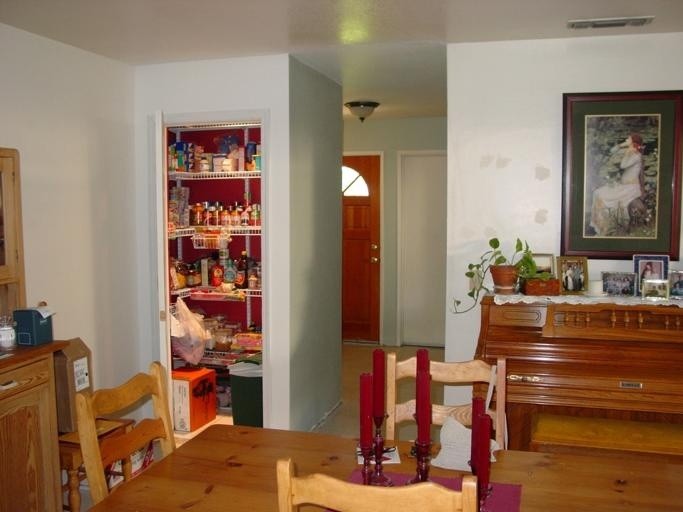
[58,417,136,512]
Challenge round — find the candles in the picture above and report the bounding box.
[418,370,430,442]
[413,349,433,425]
[373,348,385,416]
[360,373,372,446]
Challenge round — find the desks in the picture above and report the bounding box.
[83,425,683,512]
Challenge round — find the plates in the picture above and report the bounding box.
[582,291,609,297]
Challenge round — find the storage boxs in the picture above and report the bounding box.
[228,351,262,427]
[522,278,559,296]
[14,308,52,346]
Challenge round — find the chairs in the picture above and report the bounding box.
[386,353,505,451]
[76,362,191,505]
[276,458,477,512]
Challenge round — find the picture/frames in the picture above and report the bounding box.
[600,271,637,297]
[633,254,669,296]
[556,256,588,295]
[668,271,683,299]
[560,90,683,261]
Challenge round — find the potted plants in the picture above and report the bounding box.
[449,238,555,314]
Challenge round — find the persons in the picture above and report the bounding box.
[590,135,646,237]
[677,276,683,289]
[571,264,582,291]
[561,265,574,291]
[641,261,656,290]
[605,274,632,295]
[671,282,683,295]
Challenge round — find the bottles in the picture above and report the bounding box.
[199,157,210,171]
[232,202,240,209]
[226,322,241,337]
[202,209,212,225]
[248,322,257,333]
[219,248,229,267]
[171,259,186,290]
[236,206,244,212]
[207,206,216,213]
[218,206,226,211]
[227,206,236,211]
[212,210,221,225]
[220,210,231,225]
[193,203,203,225]
[204,319,218,350]
[215,329,232,352]
[230,210,240,226]
[224,260,236,283]
[213,201,222,207]
[189,206,194,226]
[235,251,247,289]
[211,314,227,329]
[188,269,198,287]
[251,155,261,171]
[250,205,260,225]
[240,211,250,226]
[244,192,251,208]
[204,200,210,209]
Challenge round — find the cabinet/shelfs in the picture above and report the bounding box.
[472,293,683,451]
[0,340,70,511]
[168,124,261,410]
[172,367,216,431]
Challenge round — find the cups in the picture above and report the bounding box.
[587,280,603,294]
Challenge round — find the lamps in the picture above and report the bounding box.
[344,101,380,123]
